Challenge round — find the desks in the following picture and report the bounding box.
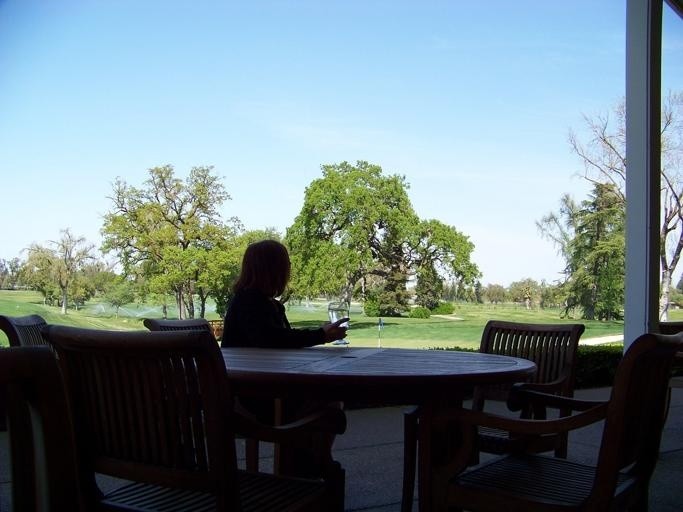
[204,338,536,512]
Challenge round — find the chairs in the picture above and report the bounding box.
[436,327,683,509]
[43,324,206,482]
[3,342,95,510]
[400,317,586,511]
[1,311,55,350]
[140,312,348,486]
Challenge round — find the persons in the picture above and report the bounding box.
[219,239,350,480]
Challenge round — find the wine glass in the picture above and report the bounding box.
[328,301,350,345]
